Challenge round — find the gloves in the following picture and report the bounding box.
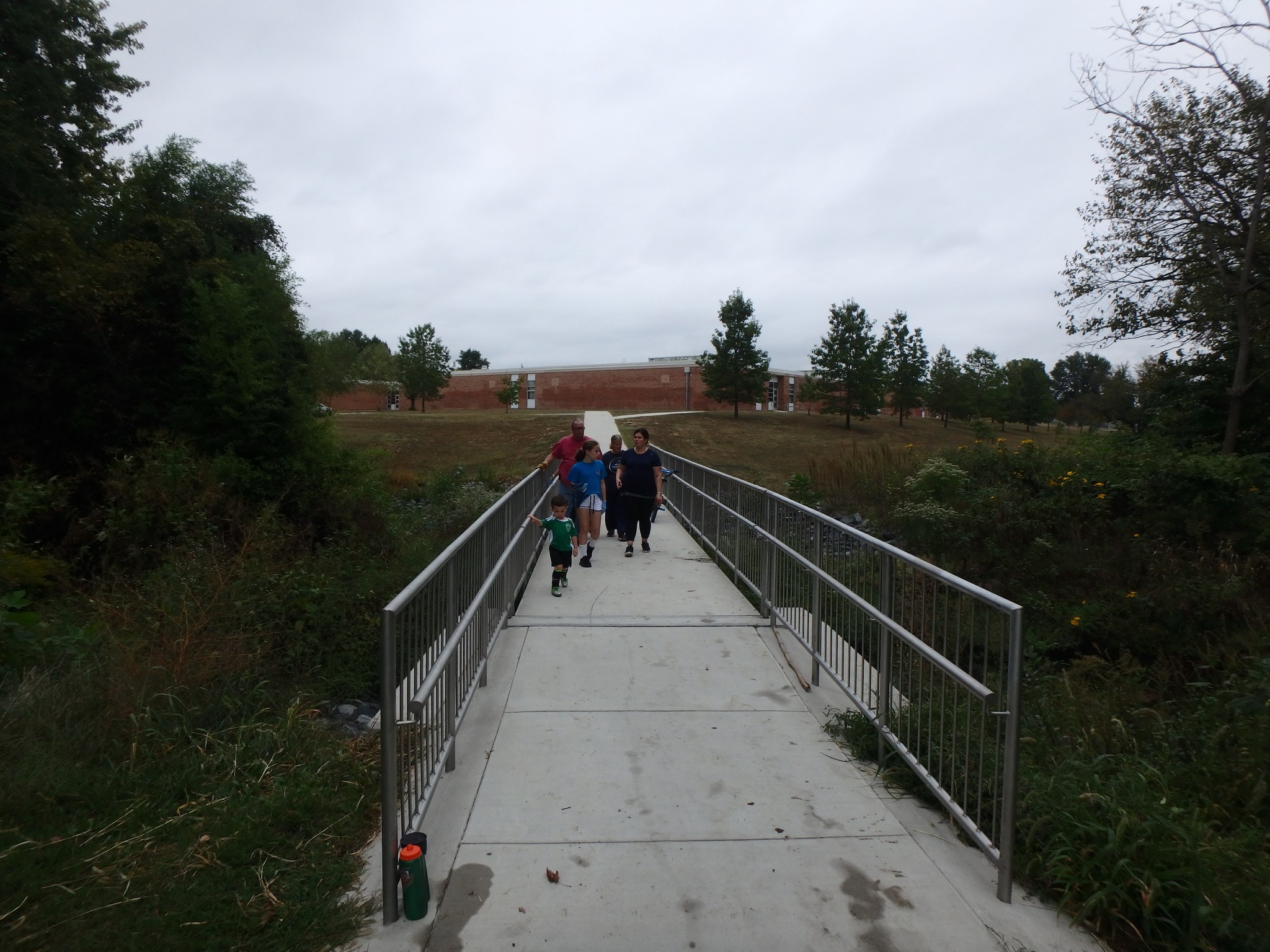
[536,461,549,473]
[569,482,587,489]
[601,501,606,515]
[548,478,554,487]
[580,483,588,492]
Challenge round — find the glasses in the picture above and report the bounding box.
[572,426,585,431]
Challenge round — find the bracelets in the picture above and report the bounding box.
[656,492,662,495]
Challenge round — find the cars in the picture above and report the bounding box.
[310,402,332,418]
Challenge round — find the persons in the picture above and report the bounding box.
[535,418,603,548]
[526,495,579,597]
[616,427,662,557]
[603,434,625,541]
[566,439,607,567]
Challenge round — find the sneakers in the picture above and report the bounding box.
[617,529,625,540]
[641,541,651,552]
[560,576,568,587]
[607,529,615,537]
[624,543,634,557]
[587,541,595,560]
[579,556,592,568]
[551,586,562,597]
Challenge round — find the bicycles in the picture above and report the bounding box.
[651,469,679,523]
